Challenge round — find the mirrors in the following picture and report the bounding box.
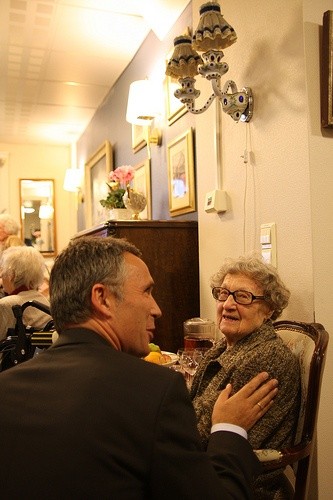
[17,177,58,257]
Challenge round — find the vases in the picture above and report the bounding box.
[108,208,128,220]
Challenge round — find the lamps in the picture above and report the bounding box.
[164,0,253,123]
[122,81,160,156]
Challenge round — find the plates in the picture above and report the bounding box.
[156,350,180,368]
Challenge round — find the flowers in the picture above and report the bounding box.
[100,163,134,208]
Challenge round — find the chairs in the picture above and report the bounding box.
[254,320,330,500]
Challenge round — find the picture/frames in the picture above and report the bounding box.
[322,9,333,128]
[127,50,196,221]
[84,138,113,228]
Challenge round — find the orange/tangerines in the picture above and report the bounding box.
[142,352,164,365]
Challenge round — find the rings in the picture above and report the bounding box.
[256,402,264,409]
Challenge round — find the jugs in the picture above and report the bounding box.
[183,317,215,354]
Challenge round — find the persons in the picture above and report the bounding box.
[0,236,279,500]
[189,255,302,500]
[0,216,53,341]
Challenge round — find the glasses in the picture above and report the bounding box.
[212,286,265,305]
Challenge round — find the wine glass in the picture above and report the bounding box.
[176,348,203,391]
